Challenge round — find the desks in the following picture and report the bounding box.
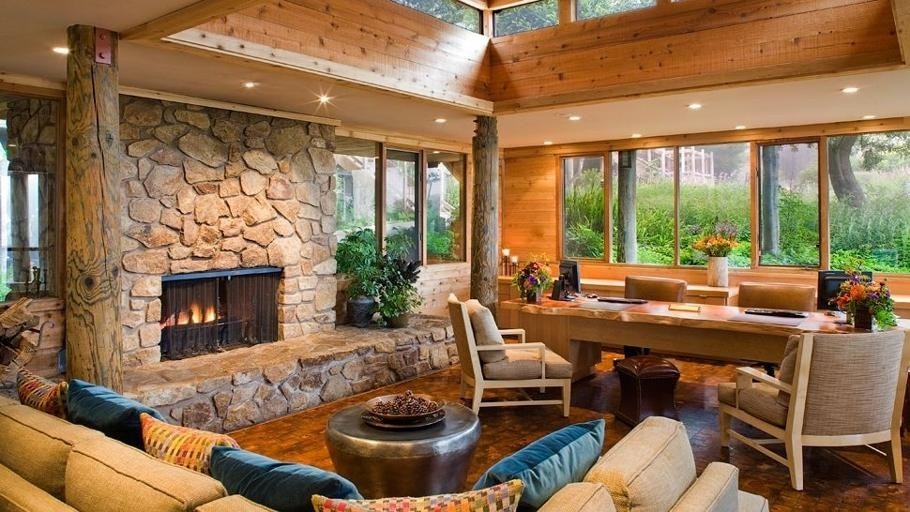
[500,290,910,385]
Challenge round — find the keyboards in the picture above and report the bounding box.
[600,297,648,304]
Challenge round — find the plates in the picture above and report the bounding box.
[360,394,447,432]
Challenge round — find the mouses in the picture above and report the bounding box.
[587,293,598,300]
[746,308,810,318]
[825,311,836,317]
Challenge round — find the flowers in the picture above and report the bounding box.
[829,268,897,330]
[513,259,552,303]
[693,237,738,257]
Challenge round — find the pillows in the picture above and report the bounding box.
[69,379,166,450]
[473,418,605,512]
[210,447,364,512]
[139,412,242,476]
[311,479,526,512]
[17,368,69,418]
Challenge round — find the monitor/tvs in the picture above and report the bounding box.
[549,259,581,303]
[818,269,874,325]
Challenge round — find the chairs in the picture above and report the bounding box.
[738,282,818,312]
[448,293,573,417]
[623,274,686,303]
[718,329,909,490]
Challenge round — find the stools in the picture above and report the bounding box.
[613,354,680,427]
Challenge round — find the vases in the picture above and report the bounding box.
[854,304,872,330]
[707,257,729,288]
[526,289,542,304]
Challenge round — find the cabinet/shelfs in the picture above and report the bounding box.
[496,275,739,364]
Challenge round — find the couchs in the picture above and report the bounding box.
[0,392,279,512]
[537,414,769,512]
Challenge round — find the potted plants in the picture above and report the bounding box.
[378,268,421,328]
[346,266,378,326]
